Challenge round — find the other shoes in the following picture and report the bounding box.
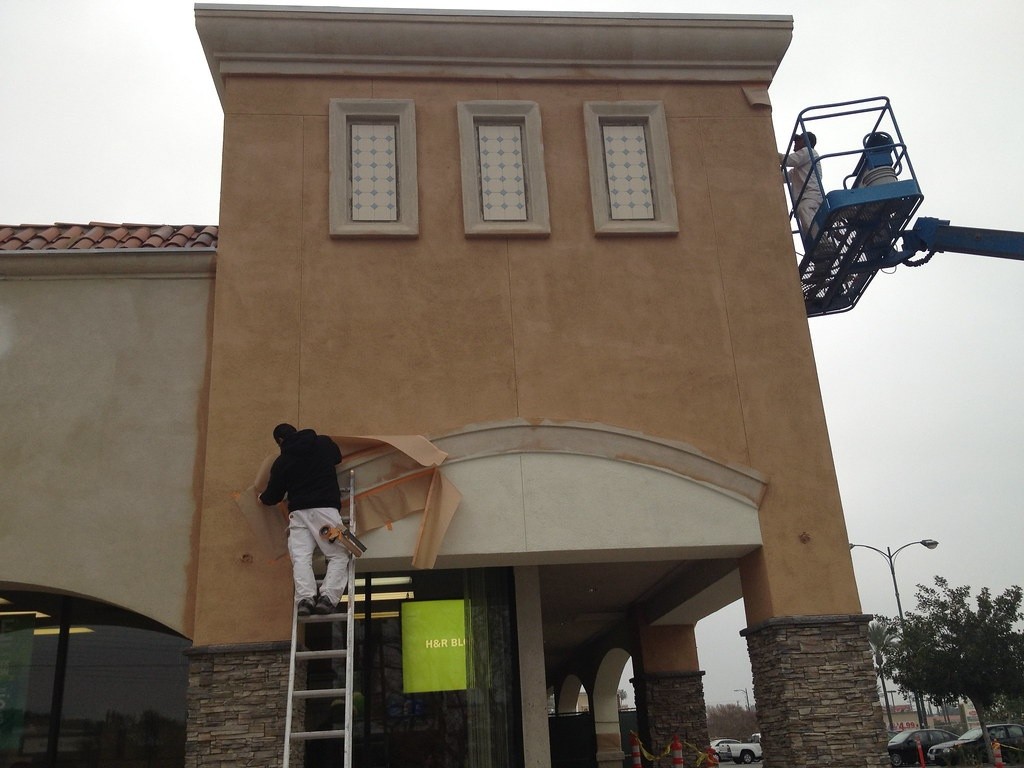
[297,599,315,615]
[316,595,335,614]
[803,262,831,284]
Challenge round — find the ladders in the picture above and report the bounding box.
[283,470,354,768]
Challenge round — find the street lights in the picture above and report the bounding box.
[849,540,940,729]
[886,690,897,713]
[733,688,750,711]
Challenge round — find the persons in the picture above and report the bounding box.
[778,132,834,274]
[256,423,352,617]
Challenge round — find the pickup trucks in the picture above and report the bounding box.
[719,733,763,764]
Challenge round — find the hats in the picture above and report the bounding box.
[793,131,816,147]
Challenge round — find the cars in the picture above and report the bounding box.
[710,739,742,762]
[887,729,960,768]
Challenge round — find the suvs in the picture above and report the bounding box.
[927,724,1024,768]
